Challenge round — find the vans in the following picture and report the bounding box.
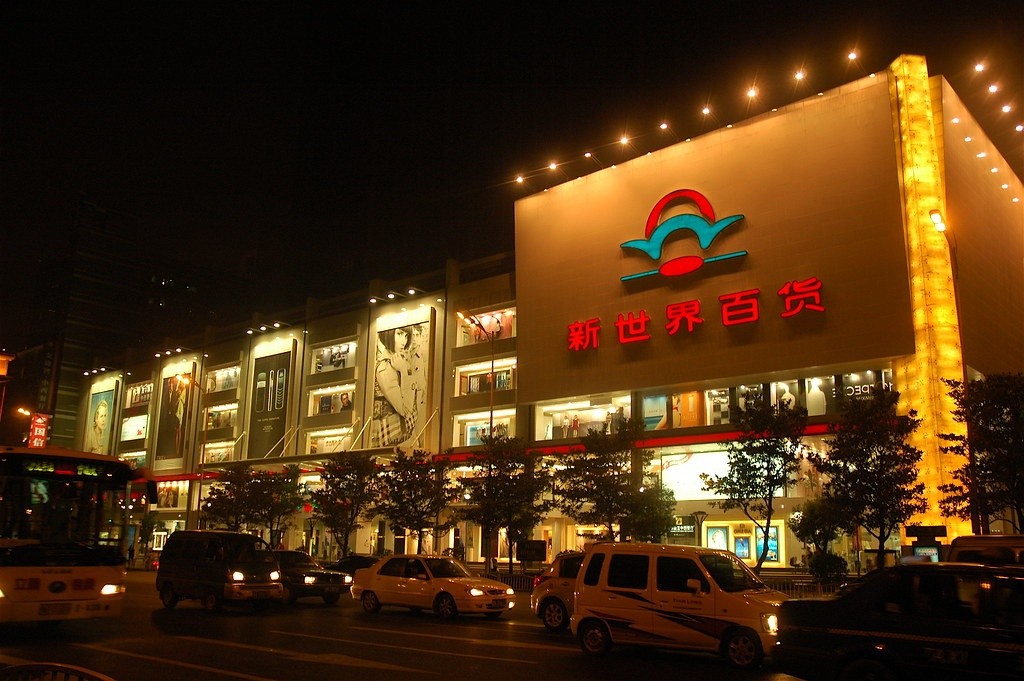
[945,530,1024,604]
[568,539,792,672]
[153,527,284,615]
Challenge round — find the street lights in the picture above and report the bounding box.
[176,373,217,530]
[454,307,502,574]
[304,517,321,556]
[690,511,708,546]
[428,502,446,555]
[18,405,56,447]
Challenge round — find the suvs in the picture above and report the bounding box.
[528,551,584,631]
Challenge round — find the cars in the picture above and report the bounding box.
[324,555,383,592]
[774,558,1024,681]
[240,549,353,607]
[348,553,516,623]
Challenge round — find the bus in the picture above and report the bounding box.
[0,447,161,624]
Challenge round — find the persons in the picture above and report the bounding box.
[85,400,108,454]
[145,544,150,556]
[225,371,238,389]
[606,411,612,435]
[561,416,569,438]
[806,547,819,560]
[170,374,187,457]
[476,426,486,439]
[371,327,418,448]
[486,372,493,391]
[158,485,179,508]
[571,415,580,438]
[340,393,352,410]
[128,545,135,564]
